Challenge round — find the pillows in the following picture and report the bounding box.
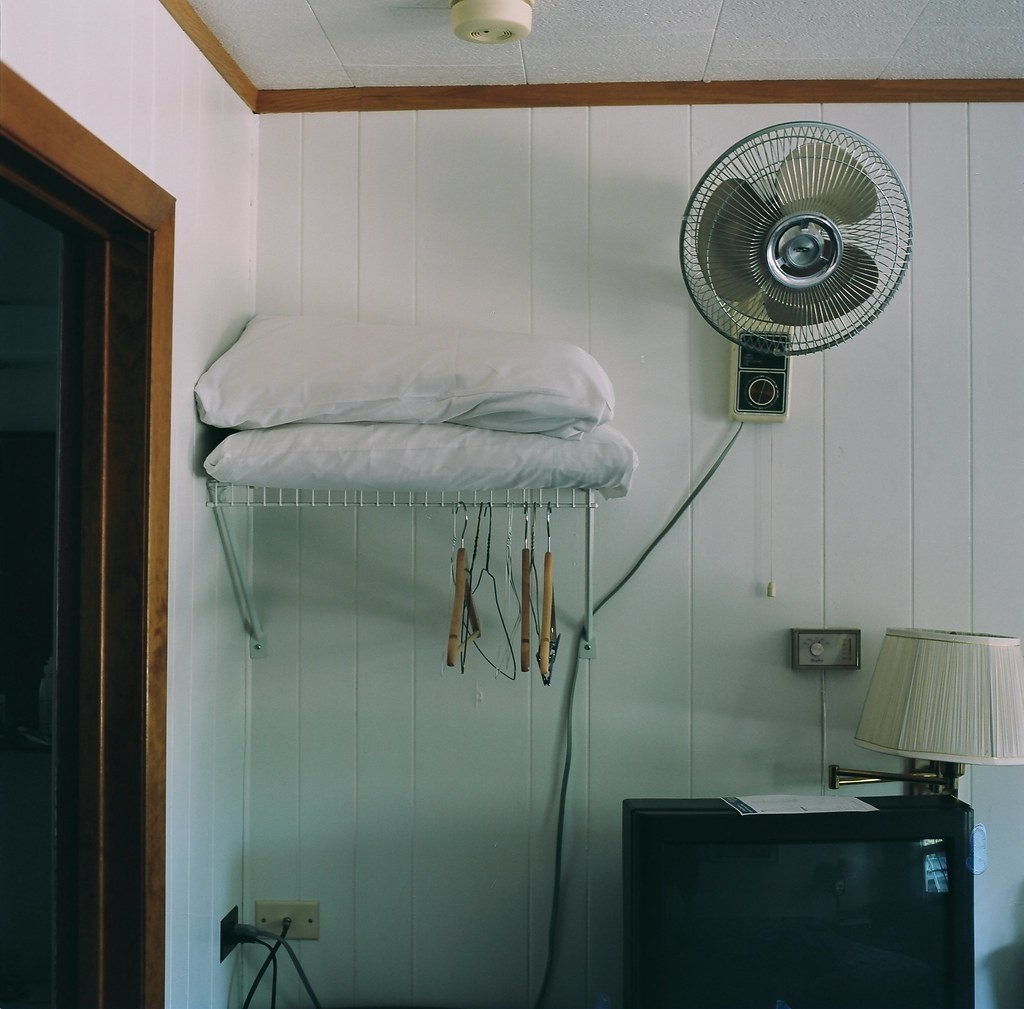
[203,426,639,500]
[195,310,615,444]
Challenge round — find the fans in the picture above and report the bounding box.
[673,119,914,425]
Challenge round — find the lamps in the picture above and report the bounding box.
[829,623,1024,801]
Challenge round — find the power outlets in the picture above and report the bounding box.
[255,900,320,943]
[220,906,240,962]
